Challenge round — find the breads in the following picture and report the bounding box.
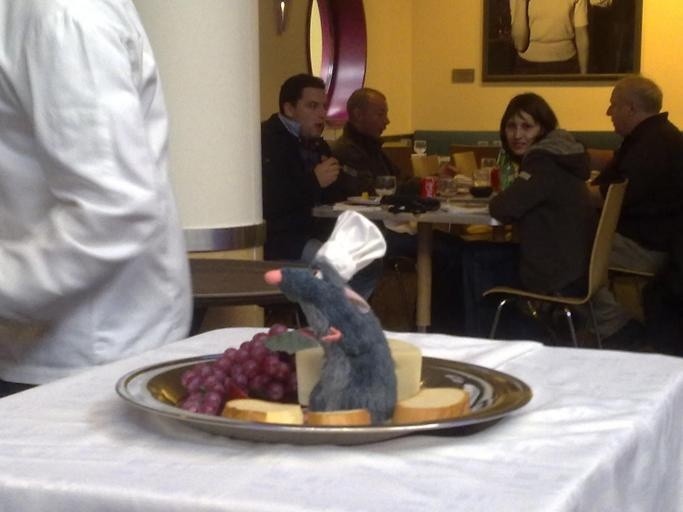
[221,397,308,428]
[304,407,371,428]
[391,385,469,425]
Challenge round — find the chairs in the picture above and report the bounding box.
[609,234,660,334]
[453,150,477,172]
[413,153,442,175]
[586,146,615,172]
[479,176,630,348]
[379,145,417,180]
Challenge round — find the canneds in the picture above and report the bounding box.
[420,176,437,200]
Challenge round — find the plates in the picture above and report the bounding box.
[349,196,381,206]
[114,353,535,447]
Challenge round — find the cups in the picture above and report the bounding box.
[482,158,497,168]
[374,175,396,195]
[470,168,492,187]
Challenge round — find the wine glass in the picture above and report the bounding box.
[413,139,428,155]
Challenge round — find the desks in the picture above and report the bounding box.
[312,165,508,335]
[452,138,505,161]
[186,260,331,336]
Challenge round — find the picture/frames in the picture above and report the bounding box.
[480,1,643,86]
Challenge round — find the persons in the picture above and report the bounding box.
[0,0,195,396]
[327,85,412,299]
[509,1,591,73]
[597,75,680,274]
[257,73,360,277]
[448,91,593,335]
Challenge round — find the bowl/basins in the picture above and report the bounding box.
[468,185,493,197]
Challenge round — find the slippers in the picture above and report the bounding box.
[591,317,634,342]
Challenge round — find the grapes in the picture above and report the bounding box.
[180,321,318,418]
